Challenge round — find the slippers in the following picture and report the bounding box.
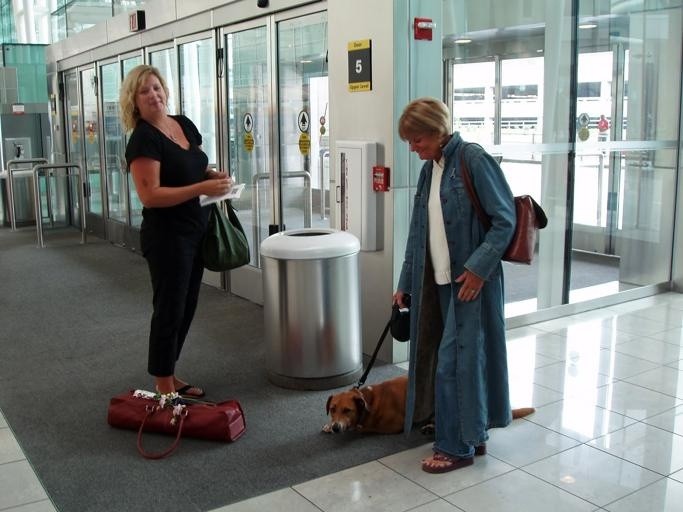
[175,384,206,400]
[421,452,474,474]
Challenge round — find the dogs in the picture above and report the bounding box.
[322,375,537,433]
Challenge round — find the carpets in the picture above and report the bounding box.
[0,223,434,512]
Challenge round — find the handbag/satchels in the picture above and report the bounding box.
[202,199,250,271]
[500,194,548,266]
[107,388,247,444]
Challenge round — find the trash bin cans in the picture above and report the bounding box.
[0,167,36,226]
[260,227,362,391]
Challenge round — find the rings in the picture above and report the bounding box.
[470,287,476,294]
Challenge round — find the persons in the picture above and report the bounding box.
[119,64,232,399]
[393,98,517,474]
